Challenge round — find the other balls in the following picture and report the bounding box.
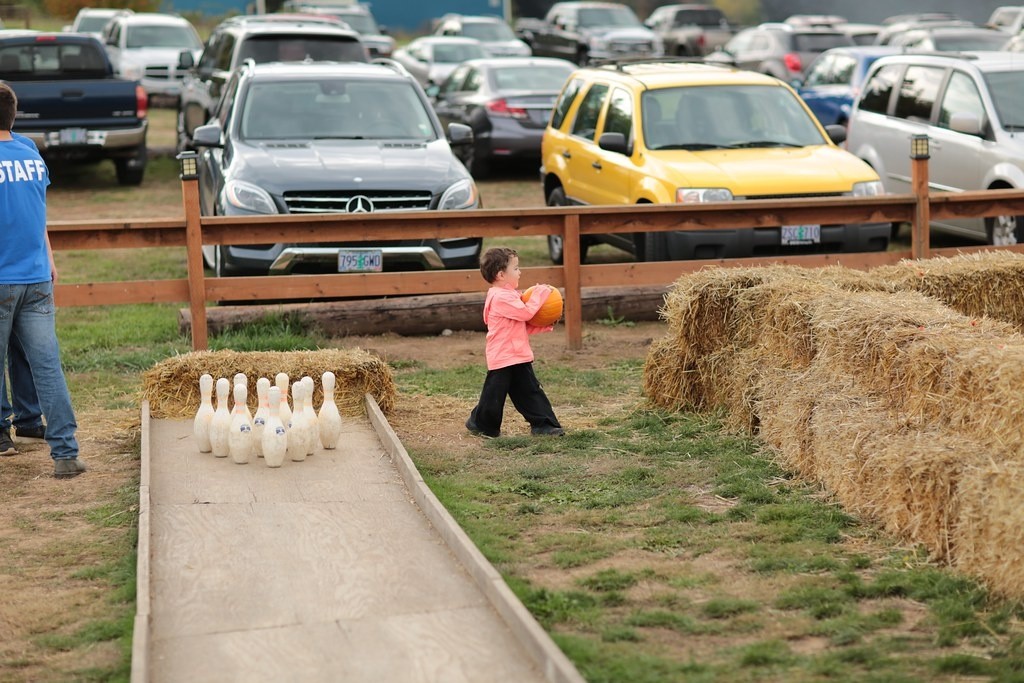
[522,284,564,328]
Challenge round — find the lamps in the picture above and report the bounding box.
[176,150,200,180]
[908,133,930,159]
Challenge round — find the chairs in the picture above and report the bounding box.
[59,54,83,69]
[645,95,679,146]
[0,53,22,72]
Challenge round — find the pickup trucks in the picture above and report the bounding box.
[1,30,148,185]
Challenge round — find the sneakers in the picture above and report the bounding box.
[531,425,565,437]
[15,425,48,444]
[0,428,19,456]
[465,417,500,440]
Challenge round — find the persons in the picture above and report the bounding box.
[0,80,86,478]
[465,247,566,438]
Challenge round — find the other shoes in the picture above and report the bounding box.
[53,456,85,478]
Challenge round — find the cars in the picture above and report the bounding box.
[840,7,1024,53]
[62,7,134,57]
[546,2,664,67]
[392,36,488,89]
[425,58,582,180]
[780,46,906,145]
[642,4,732,56]
[301,4,395,65]
[176,14,372,151]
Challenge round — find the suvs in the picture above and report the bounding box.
[99,13,206,104]
[432,13,532,58]
[193,57,484,305]
[846,52,1024,247]
[703,22,855,82]
[540,56,892,261]
